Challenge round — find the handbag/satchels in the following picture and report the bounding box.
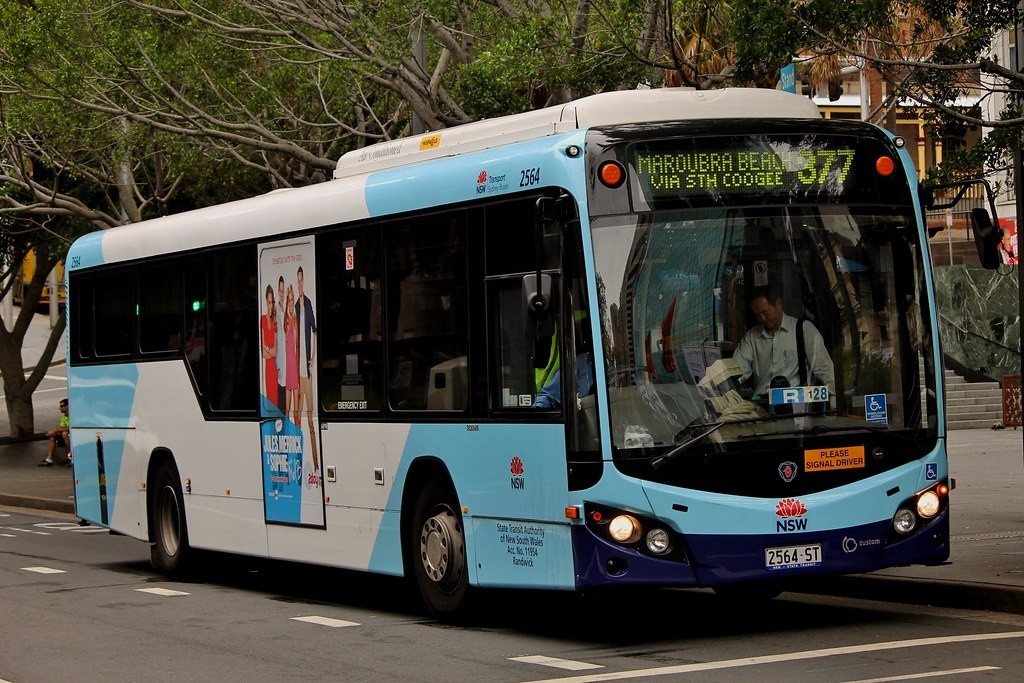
[805,376,828,415]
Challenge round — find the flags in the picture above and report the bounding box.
[659,297,676,373]
[643,331,657,379]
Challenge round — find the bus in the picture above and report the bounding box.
[66,88,1005,636]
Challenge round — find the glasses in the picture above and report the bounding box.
[60,405,66,408]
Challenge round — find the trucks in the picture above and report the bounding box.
[10,243,66,315]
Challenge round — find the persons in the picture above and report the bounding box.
[259,264,321,473]
[535,319,626,411]
[37,398,72,467]
[729,289,837,416]
[1000,225,1013,262]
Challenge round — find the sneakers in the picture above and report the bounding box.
[65,458,73,467]
[37,458,54,467]
